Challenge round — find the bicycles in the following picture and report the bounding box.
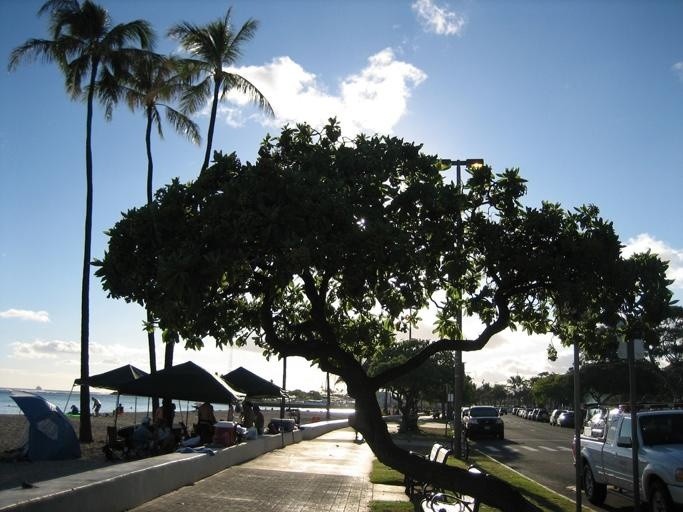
[447,418,469,460]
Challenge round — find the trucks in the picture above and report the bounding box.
[573,405,682,511]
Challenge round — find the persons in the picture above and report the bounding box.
[71,397,265,456]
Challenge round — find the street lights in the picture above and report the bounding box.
[436,155,486,462]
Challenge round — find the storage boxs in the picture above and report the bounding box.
[271,418,295,432]
[213,424,237,448]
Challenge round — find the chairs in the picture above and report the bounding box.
[405,443,453,503]
[414,464,485,512]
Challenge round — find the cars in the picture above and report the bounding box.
[459,402,573,440]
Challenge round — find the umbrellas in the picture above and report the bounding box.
[10,389,81,457]
